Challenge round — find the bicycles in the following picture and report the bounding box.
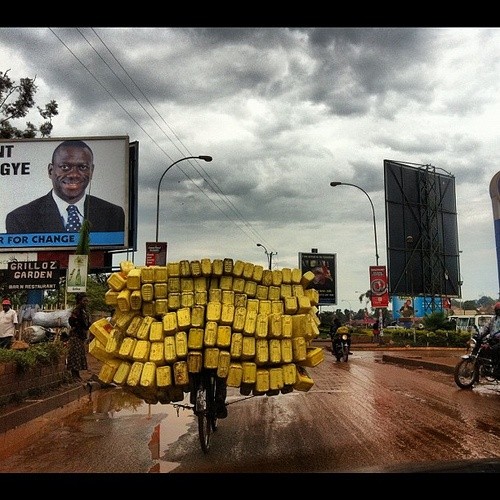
[187,367,236,455]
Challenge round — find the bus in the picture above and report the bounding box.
[455,313,495,333]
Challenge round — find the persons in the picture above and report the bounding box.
[373,320,379,344]
[399,300,413,318]
[480,302,500,381]
[68,294,90,377]
[0,300,17,349]
[331,317,353,355]
[6,141,126,231]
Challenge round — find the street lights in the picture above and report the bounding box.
[256,243,277,271]
[151,155,213,242]
[329,181,379,266]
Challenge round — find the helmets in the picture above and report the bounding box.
[2,300,12,305]
[493,301,500,310]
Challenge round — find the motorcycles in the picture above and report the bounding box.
[333,326,356,364]
[455,325,500,390]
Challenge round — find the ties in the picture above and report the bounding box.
[65,205,83,233]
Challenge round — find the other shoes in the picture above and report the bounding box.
[71,370,83,383]
